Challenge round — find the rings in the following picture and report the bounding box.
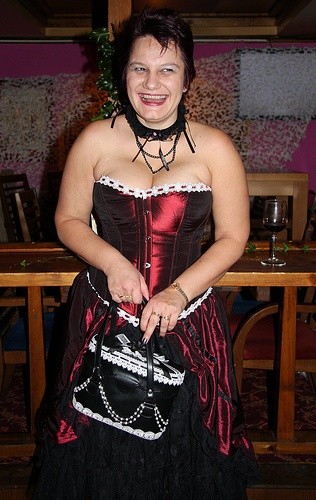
[125,295,133,300]
[119,296,125,300]
[161,315,170,320]
[152,312,161,318]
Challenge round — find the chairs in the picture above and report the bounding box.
[0,172,316,398]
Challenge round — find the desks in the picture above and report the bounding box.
[0,250,316,459]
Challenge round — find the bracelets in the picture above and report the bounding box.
[171,282,188,303]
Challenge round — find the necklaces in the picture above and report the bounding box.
[124,104,187,173]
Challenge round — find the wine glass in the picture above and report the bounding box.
[261,200,288,266]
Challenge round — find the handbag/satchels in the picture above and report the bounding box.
[71,295,185,440]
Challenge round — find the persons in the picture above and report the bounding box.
[26,13,250,500]
